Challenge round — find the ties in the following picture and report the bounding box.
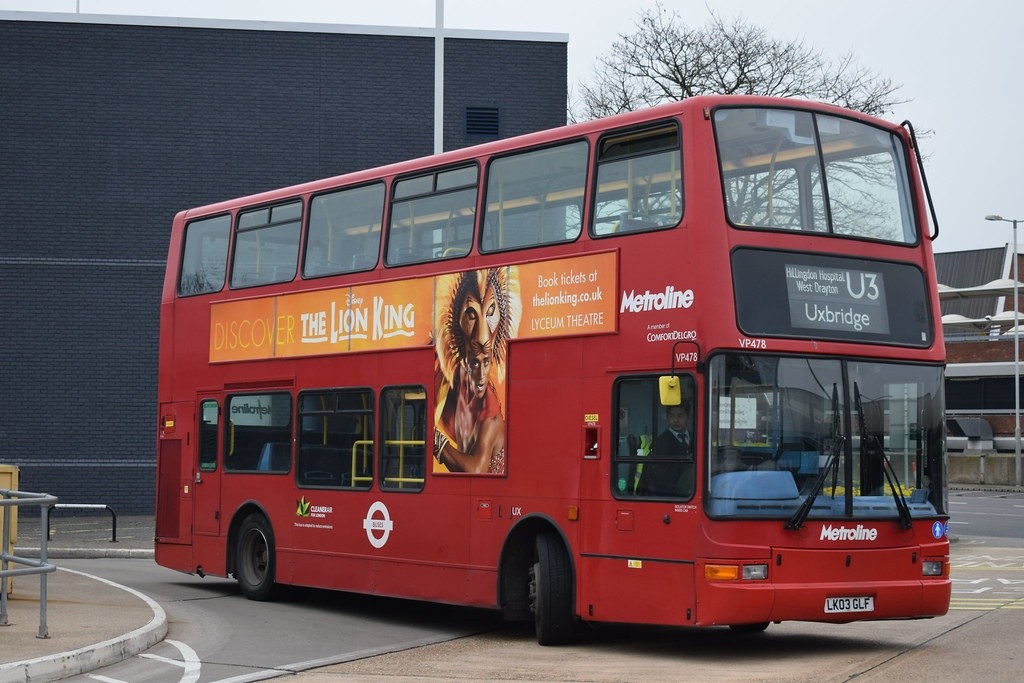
[677,433,689,447]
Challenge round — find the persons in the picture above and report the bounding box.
[433,349,505,474]
[655,398,693,498]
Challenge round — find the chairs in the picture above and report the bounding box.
[203,276,225,291]
[352,253,377,269]
[661,215,681,226]
[620,212,659,233]
[396,247,426,263]
[273,265,296,282]
[341,472,371,488]
[304,471,334,486]
[312,262,341,275]
[240,272,268,286]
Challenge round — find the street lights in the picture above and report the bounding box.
[985,214,1023,488]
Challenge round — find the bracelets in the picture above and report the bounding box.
[434,426,449,464]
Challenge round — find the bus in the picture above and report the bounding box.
[153,95,953,646]
